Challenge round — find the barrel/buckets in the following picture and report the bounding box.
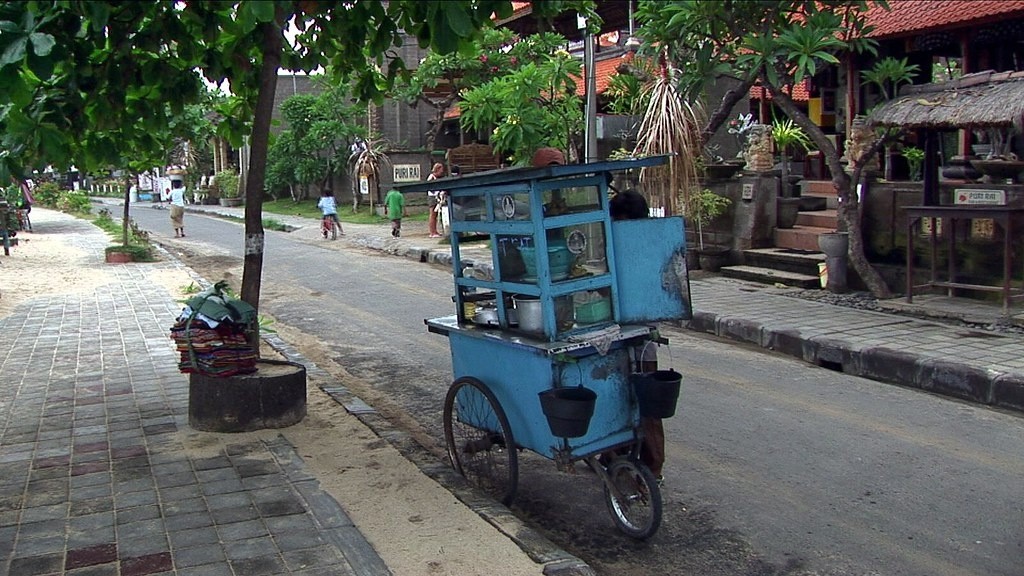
[818,263,828,287]
[629,337,683,418]
[491,242,527,278]
[537,357,598,438]
[871,264,897,292]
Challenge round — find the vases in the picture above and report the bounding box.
[705,163,739,180]
[725,160,746,170]
[818,232,849,292]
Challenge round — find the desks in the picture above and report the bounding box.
[898,205,1024,311]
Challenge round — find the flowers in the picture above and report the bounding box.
[728,112,760,158]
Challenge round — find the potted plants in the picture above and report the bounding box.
[215,169,243,207]
[677,188,709,270]
[770,103,817,229]
[688,190,733,272]
[594,54,655,142]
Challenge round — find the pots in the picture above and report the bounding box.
[511,292,575,336]
[517,239,573,274]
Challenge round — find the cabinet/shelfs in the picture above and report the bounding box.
[446,175,619,340]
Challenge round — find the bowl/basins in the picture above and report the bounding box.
[702,165,739,178]
[724,158,747,168]
[471,308,519,325]
[940,273,993,299]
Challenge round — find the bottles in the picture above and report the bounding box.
[462,263,476,319]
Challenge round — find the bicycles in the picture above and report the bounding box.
[315,206,336,240]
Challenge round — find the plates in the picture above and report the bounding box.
[521,272,568,282]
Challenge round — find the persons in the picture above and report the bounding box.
[385,182,409,236]
[597,189,664,496]
[427,162,461,240]
[317,187,346,237]
[166,180,186,237]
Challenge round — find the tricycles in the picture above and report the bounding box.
[394,151,692,540]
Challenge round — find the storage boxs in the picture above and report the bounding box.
[954,189,1017,206]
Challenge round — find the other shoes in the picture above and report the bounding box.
[391,228,401,237]
[429,233,442,238]
